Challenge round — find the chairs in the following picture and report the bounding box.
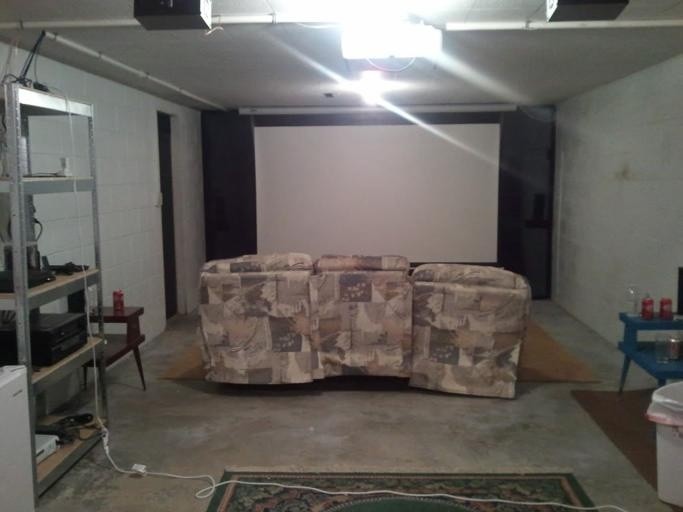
[411,263,531,399]
[196,252,315,390]
[313,256,413,380]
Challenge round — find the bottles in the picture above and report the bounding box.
[111,287,125,312]
[639,292,653,319]
[658,297,674,318]
[624,282,639,317]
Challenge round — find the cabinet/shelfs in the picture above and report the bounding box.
[0,80,108,498]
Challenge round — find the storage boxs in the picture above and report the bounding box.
[0,313,87,370]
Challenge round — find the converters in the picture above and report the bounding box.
[131,464,147,477]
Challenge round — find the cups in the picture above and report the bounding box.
[655,335,670,365]
[87,284,96,315]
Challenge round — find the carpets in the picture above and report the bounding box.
[571,387,655,494]
[206,471,595,512]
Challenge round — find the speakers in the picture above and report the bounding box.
[134,0,212,30]
[546,0,629,22]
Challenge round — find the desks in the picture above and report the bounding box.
[67,289,146,397]
[617,307,683,399]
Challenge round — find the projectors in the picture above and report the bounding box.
[341,22,444,58]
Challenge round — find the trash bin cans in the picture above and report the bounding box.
[646,380,683,508]
